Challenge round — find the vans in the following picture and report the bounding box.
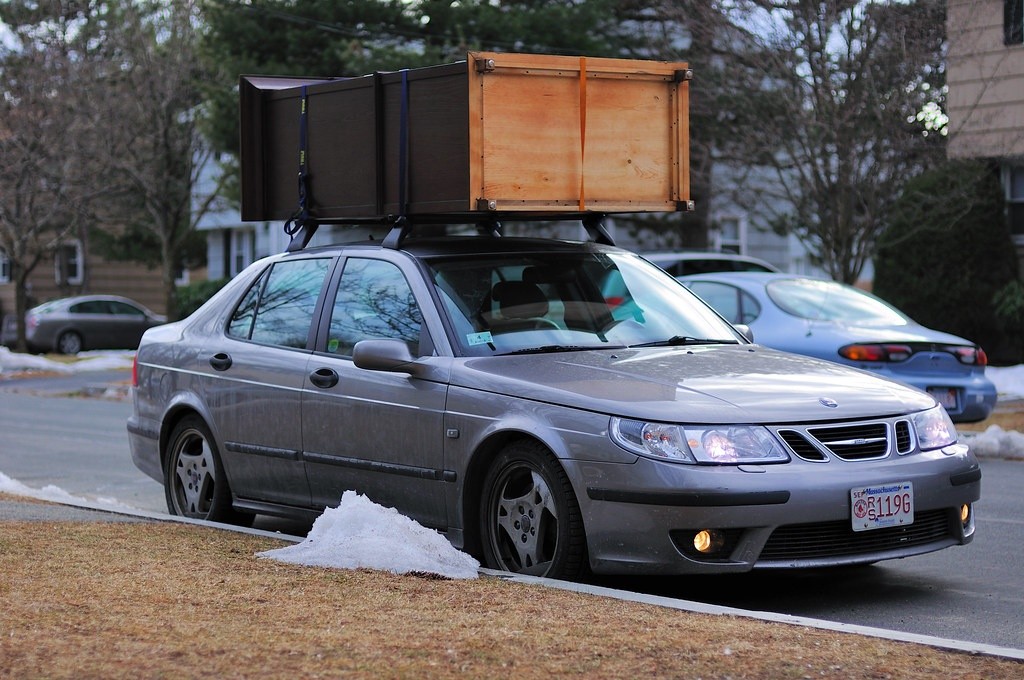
[604,254,777,313]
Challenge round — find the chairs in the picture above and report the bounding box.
[437,265,470,295]
[500,280,547,319]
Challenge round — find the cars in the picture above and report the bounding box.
[610,273,997,423]
[124,222,982,583]
[0,295,167,357]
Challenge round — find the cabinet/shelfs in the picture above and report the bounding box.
[239,51,694,221]
